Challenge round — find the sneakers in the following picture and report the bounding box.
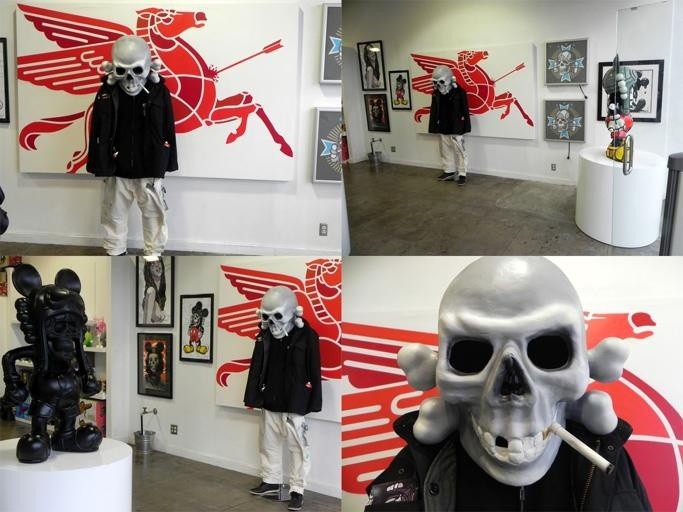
[250,482,280,494]
[288,492,304,511]
[458,176,467,185]
[437,173,456,180]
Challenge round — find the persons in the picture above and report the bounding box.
[134,256,170,326]
[243,286,323,512]
[360,41,382,90]
[427,65,471,187]
[363,255,654,512]
[84,33,180,255]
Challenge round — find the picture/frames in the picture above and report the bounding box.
[309,3,342,186]
[357,38,412,134]
[135,252,214,400]
[597,58,665,122]
[542,36,587,145]
[0,37,10,124]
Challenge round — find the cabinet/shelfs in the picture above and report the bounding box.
[8,320,105,437]
